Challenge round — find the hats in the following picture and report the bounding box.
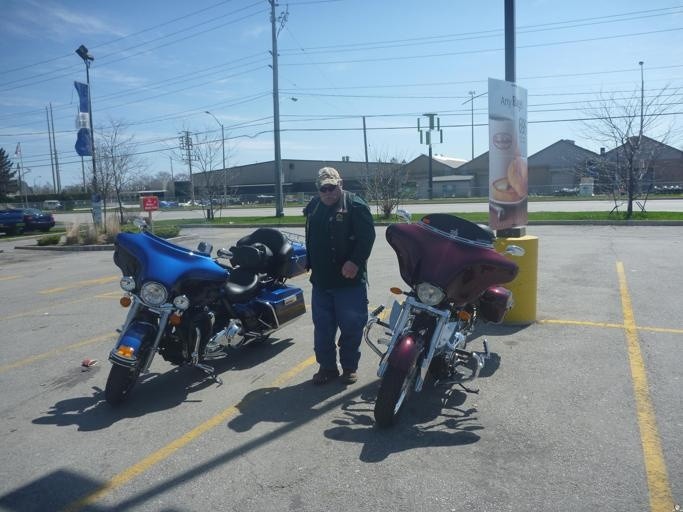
[316,167,341,186]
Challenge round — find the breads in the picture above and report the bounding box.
[507,156,528,198]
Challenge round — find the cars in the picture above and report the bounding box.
[160,197,222,209]
[0,207,54,237]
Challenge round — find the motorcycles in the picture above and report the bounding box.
[101,226,311,407]
[360,211,526,430]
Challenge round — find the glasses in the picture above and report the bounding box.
[320,185,336,192]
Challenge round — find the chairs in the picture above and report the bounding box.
[234,226,293,280]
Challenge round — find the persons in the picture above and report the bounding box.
[304,166,376,384]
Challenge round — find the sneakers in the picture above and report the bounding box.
[342,369,358,383]
[314,368,339,383]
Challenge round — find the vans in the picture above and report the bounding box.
[42,200,62,210]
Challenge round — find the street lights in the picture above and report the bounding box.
[202,111,227,209]
[636,59,648,138]
[75,45,103,230]
[279,96,297,106]
[467,91,477,173]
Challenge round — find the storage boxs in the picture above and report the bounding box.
[256,285,307,327]
[478,286,513,324]
[290,245,307,277]
